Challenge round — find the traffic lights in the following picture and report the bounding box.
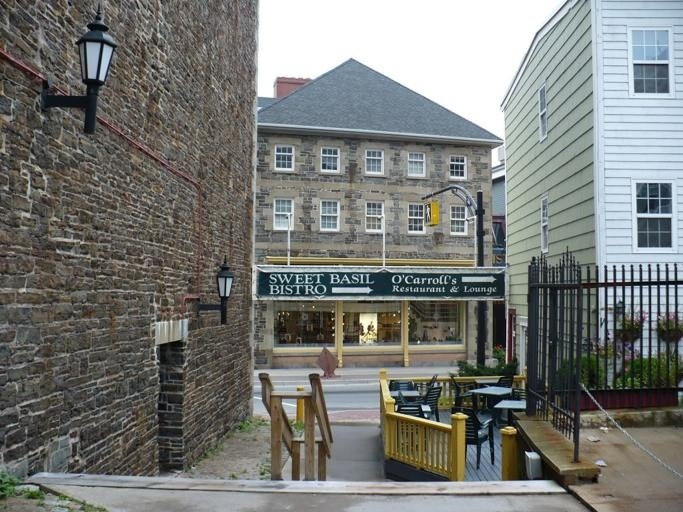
[423,200,440,225]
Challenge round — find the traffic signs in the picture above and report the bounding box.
[249,262,503,305]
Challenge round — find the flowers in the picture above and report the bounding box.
[616,309,648,329]
[657,312,683,329]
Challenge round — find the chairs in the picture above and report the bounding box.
[389,375,529,422]
[450,407,493,468]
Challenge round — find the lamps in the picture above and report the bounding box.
[43,1,115,133]
[198,255,233,325]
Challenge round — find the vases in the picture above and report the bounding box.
[657,328,682,341]
[615,328,638,342]
